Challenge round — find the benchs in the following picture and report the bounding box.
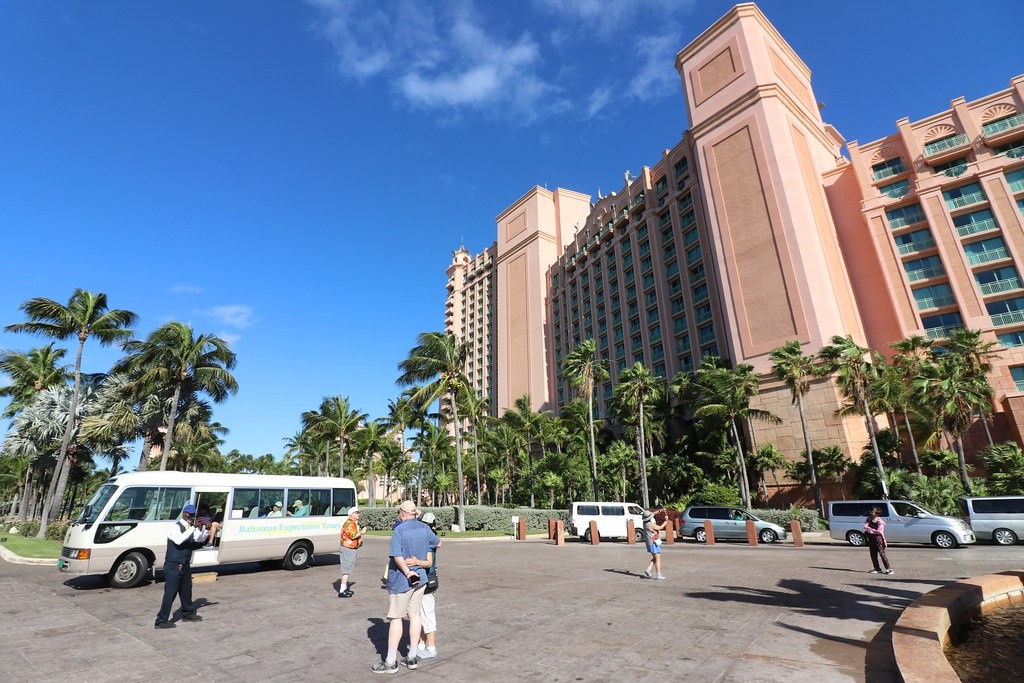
[249,505,350,519]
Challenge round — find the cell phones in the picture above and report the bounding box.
[409,574,421,584]
[200,517,209,524]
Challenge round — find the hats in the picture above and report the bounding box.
[421,512,437,522]
[291,500,303,507]
[272,501,282,507]
[182,504,198,515]
[395,500,418,515]
[641,510,654,522]
[348,507,360,516]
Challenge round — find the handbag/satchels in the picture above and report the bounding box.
[653,537,663,547]
[422,570,439,594]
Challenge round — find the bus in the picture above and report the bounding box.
[56,470,359,590]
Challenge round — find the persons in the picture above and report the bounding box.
[863,506,894,574]
[733,511,744,520]
[370,499,442,674]
[266,501,282,517]
[287,497,309,517]
[642,508,669,580]
[338,506,367,598]
[154,504,212,629]
[203,502,226,549]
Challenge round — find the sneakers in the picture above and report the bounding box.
[405,645,439,660]
[371,659,399,674]
[400,656,418,670]
[869,569,884,574]
[886,569,895,575]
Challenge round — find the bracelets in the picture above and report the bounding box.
[659,509,660,513]
[406,570,410,576]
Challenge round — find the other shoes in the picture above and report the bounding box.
[182,613,203,622]
[202,541,214,549]
[338,589,354,598]
[655,575,666,580]
[644,569,652,578]
[156,621,175,629]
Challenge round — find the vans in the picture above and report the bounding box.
[828,498,977,549]
[959,495,1024,546]
[569,502,657,544]
[679,506,787,543]
